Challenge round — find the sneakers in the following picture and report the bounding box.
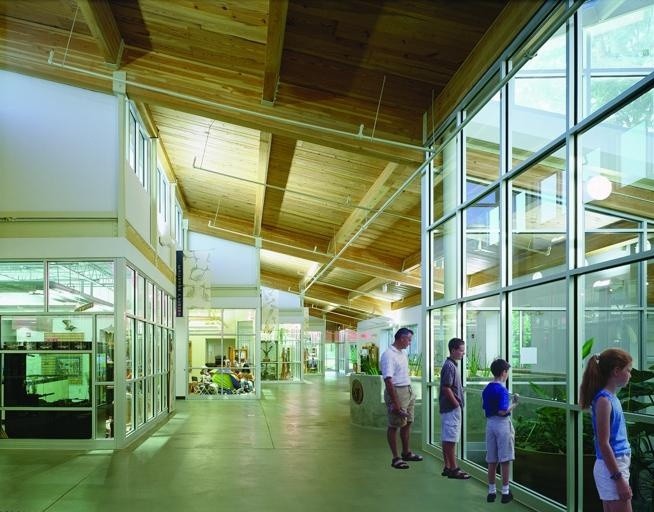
[501,492,513,503]
[487,492,497,502]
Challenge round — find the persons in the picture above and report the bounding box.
[481,358,521,504]
[379,327,423,469]
[579,347,633,511]
[438,337,472,479]
[198,355,253,395]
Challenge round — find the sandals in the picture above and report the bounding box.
[441,467,449,477]
[448,468,472,479]
[391,457,409,469]
[401,452,423,461]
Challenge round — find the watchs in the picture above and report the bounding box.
[609,468,623,481]
